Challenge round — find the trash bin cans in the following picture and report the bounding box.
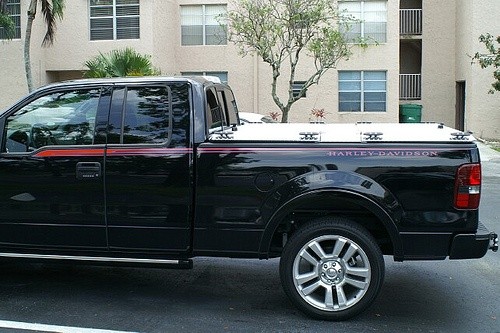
[400,102,423,123]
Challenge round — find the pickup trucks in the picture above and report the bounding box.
[0,78,500,322]
